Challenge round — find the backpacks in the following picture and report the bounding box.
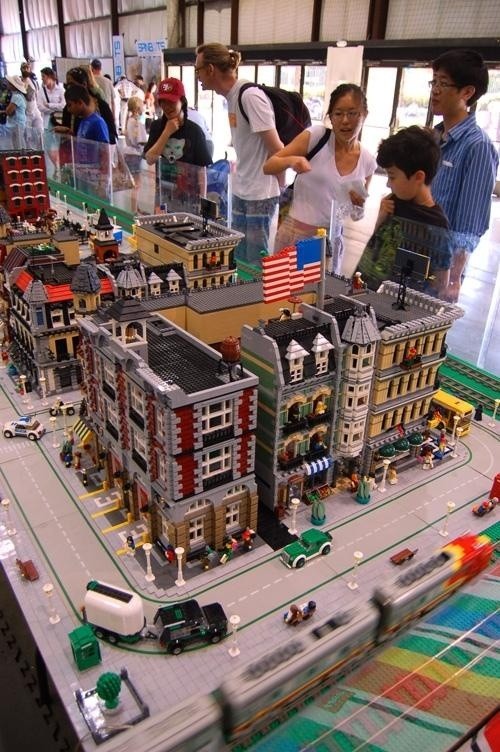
[238,80,312,148]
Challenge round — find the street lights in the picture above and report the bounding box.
[42,581,62,626]
[21,371,71,451]
[1,495,17,538]
[346,550,364,591]
[140,541,158,583]
[173,546,188,589]
[438,498,459,537]
[52,187,118,230]
[448,414,461,444]
[449,424,464,459]
[227,613,241,659]
[488,398,500,428]
[377,456,393,494]
[285,495,303,535]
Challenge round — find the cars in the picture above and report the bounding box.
[1,414,46,443]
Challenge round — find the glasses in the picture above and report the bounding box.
[331,110,365,120]
[428,80,462,91]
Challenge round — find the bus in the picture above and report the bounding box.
[430,391,474,439]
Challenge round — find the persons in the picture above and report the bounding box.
[425,49,497,303]
[1,58,211,218]
[195,43,285,267]
[354,124,452,299]
[264,84,374,273]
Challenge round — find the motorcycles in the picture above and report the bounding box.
[48,400,77,418]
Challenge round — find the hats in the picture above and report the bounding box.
[4,73,28,95]
[156,77,185,103]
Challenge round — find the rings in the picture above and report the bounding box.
[295,167,300,171]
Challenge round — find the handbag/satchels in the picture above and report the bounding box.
[51,109,65,125]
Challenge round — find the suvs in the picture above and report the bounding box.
[152,597,230,658]
[278,527,337,572]
